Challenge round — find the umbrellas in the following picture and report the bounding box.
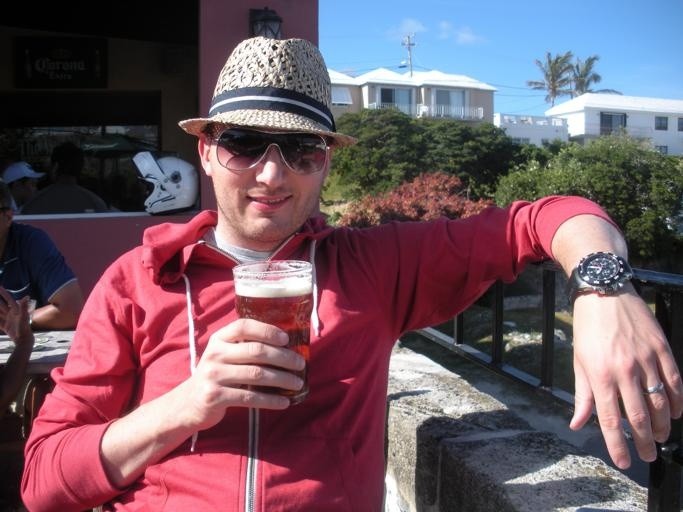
[51,131,158,159]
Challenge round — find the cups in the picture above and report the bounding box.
[232,259,315,408]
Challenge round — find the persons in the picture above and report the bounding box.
[0,285,36,409]
[18,34,682,511]
[0,160,46,213]
[0,180,85,336]
[19,140,108,214]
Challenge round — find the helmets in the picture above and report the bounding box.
[132,151,200,215]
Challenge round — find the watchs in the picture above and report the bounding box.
[562,251,642,307]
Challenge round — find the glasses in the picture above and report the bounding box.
[212,128,327,175]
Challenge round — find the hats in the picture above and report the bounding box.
[3,161,46,185]
[177,36,358,148]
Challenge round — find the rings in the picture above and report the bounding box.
[642,381,664,395]
